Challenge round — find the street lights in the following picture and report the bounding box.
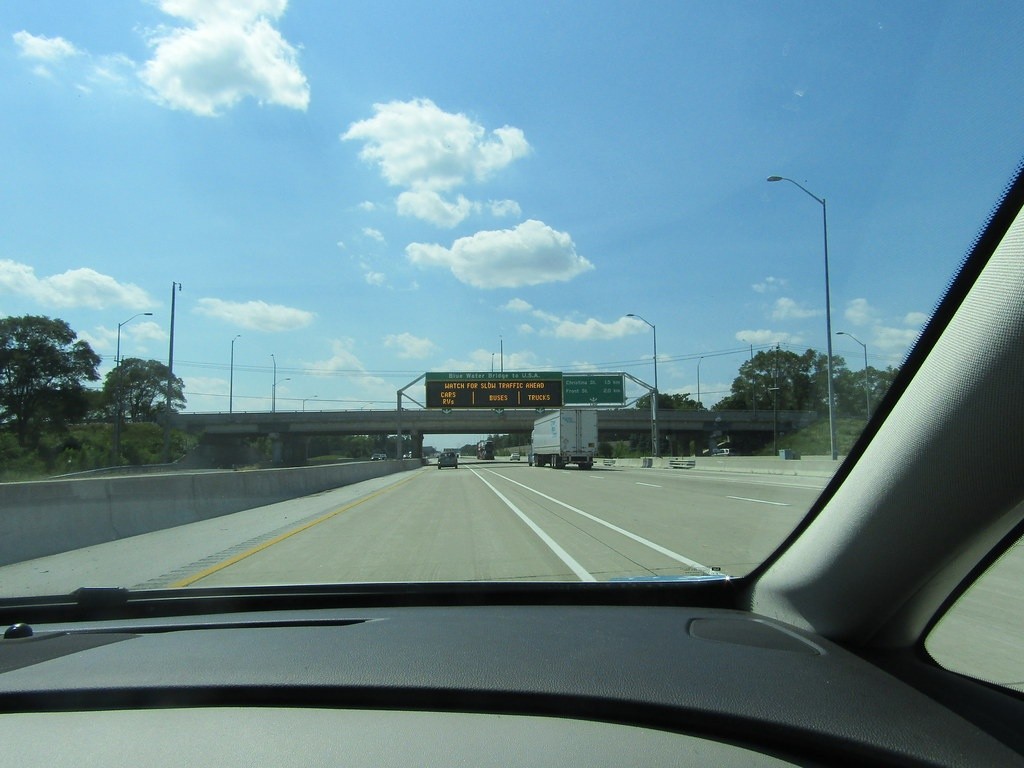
[229,333,240,415]
[116,312,153,467]
[836,331,870,420]
[627,313,658,389]
[768,176,838,462]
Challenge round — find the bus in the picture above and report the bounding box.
[477,441,493,460]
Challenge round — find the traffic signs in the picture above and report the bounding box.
[563,375,624,404]
[425,372,562,415]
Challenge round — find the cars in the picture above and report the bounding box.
[380,454,387,460]
[510,453,521,460]
[371,454,381,460]
[438,452,457,469]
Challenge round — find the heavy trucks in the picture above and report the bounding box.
[528,409,597,469]
